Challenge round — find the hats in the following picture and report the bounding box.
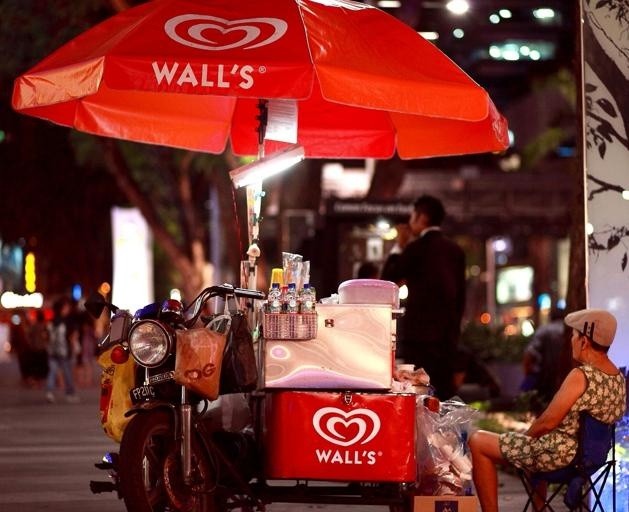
[564,308,618,346]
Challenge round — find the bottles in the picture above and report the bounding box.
[268,283,316,315]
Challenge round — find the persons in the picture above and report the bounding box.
[465,307,627,512]
[9,296,100,406]
[379,192,467,401]
[219,215,412,292]
[519,308,566,392]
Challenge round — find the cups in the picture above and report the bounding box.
[110,313,132,342]
[397,364,415,372]
[448,449,473,474]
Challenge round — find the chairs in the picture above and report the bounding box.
[515,410,616,512]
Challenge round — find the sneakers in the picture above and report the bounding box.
[44,391,85,405]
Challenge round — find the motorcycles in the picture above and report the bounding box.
[91,283,411,512]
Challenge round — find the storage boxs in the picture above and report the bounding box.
[410,496,477,512]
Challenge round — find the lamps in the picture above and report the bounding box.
[229,144,305,189]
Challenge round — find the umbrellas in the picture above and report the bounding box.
[10,1,511,291]
